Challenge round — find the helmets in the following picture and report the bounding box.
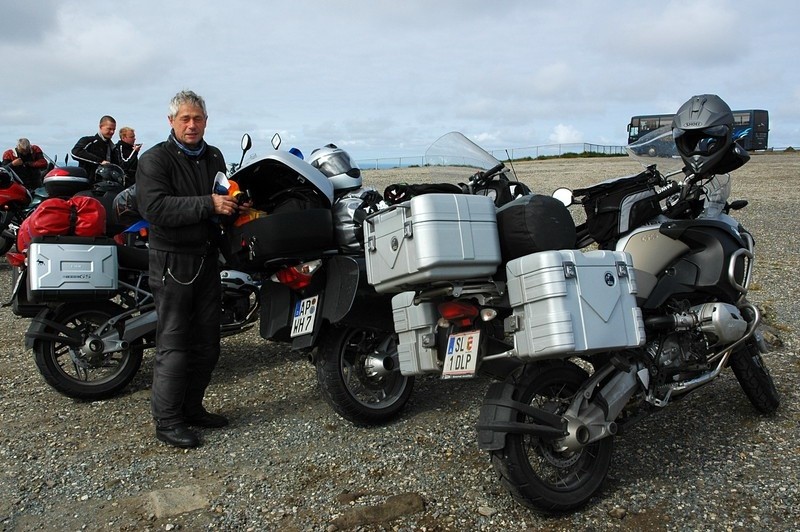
[94,164,127,186]
[670,93,735,175]
[307,145,363,191]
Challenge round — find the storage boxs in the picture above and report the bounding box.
[363,193,501,293]
[216,151,334,272]
[43,166,91,198]
[0,164,15,190]
[506,250,647,357]
[27,235,119,304]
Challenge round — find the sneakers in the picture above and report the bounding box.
[155,425,200,448]
[189,407,229,428]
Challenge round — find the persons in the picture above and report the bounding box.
[2,138,48,188]
[135,91,254,449]
[70,115,143,189]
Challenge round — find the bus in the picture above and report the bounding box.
[627,109,770,158]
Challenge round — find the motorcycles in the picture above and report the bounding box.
[0,134,387,403]
[362,127,782,519]
[221,130,534,430]
